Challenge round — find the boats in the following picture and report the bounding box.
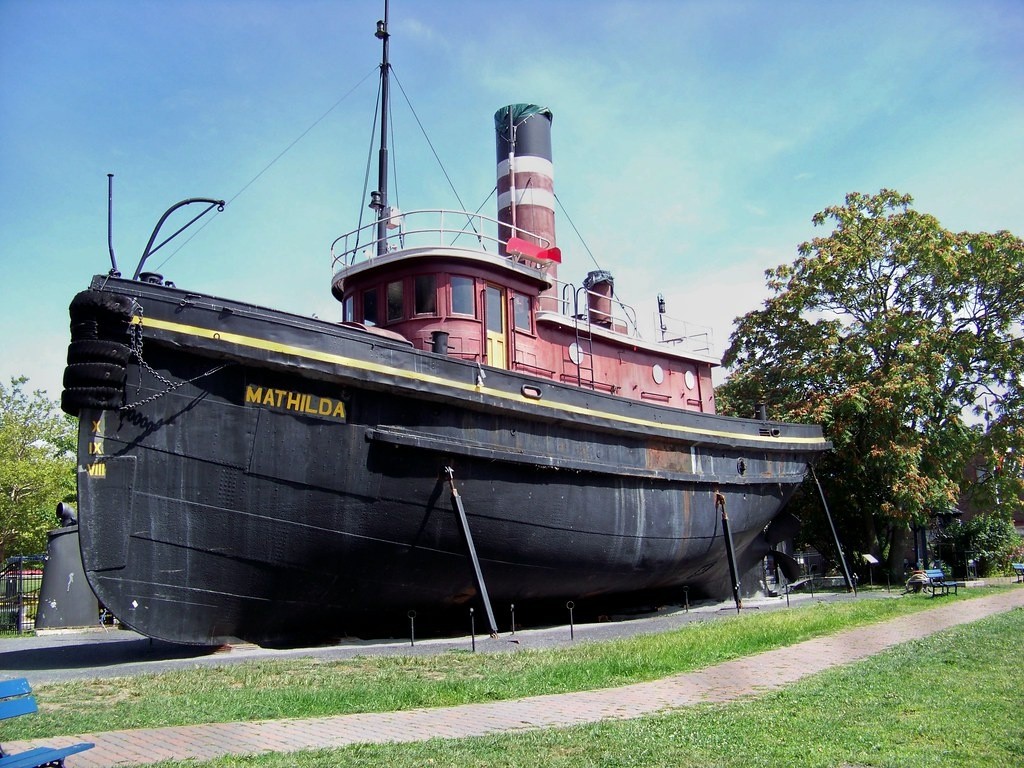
[57,1,836,648]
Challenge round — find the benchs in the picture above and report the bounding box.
[1010,563,1024,583]
[0,678,95,768]
[925,569,959,598]
